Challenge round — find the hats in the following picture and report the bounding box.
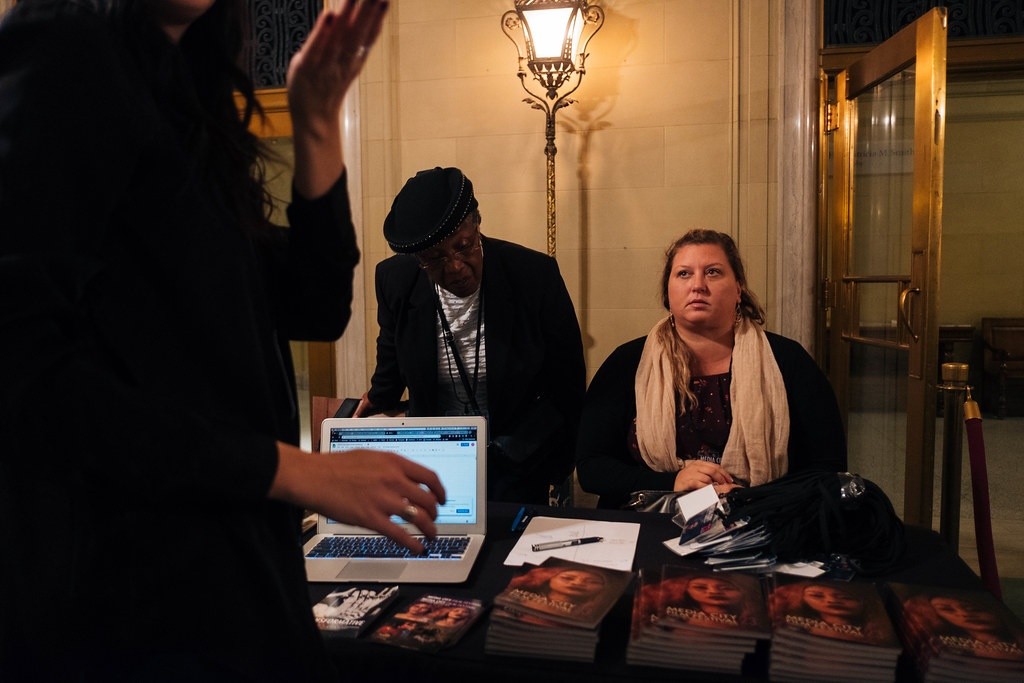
[382,167,475,257]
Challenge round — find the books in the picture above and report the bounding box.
[766,578,904,683]
[311,583,401,642]
[365,592,491,657]
[484,557,637,663]
[880,579,1024,683]
[626,564,772,676]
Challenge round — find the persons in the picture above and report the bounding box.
[575,228,848,512]
[396,565,1021,656]
[355,167,587,501]
[0,1,446,683]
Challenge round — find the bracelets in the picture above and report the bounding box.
[713,480,721,495]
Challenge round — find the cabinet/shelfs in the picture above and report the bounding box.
[825,316,1024,419]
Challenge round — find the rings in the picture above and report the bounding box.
[353,46,365,58]
[403,504,419,523]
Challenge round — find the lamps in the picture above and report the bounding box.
[500,0,606,258]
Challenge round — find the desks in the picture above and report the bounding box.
[303,501,1022,683]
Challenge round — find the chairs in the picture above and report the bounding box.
[319,398,575,507]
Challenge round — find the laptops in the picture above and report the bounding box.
[303,415,488,583]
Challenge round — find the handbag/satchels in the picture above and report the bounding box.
[725,471,904,565]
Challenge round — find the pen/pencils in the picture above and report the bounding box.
[531,535,604,552]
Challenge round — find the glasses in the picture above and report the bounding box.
[414,232,478,269]
